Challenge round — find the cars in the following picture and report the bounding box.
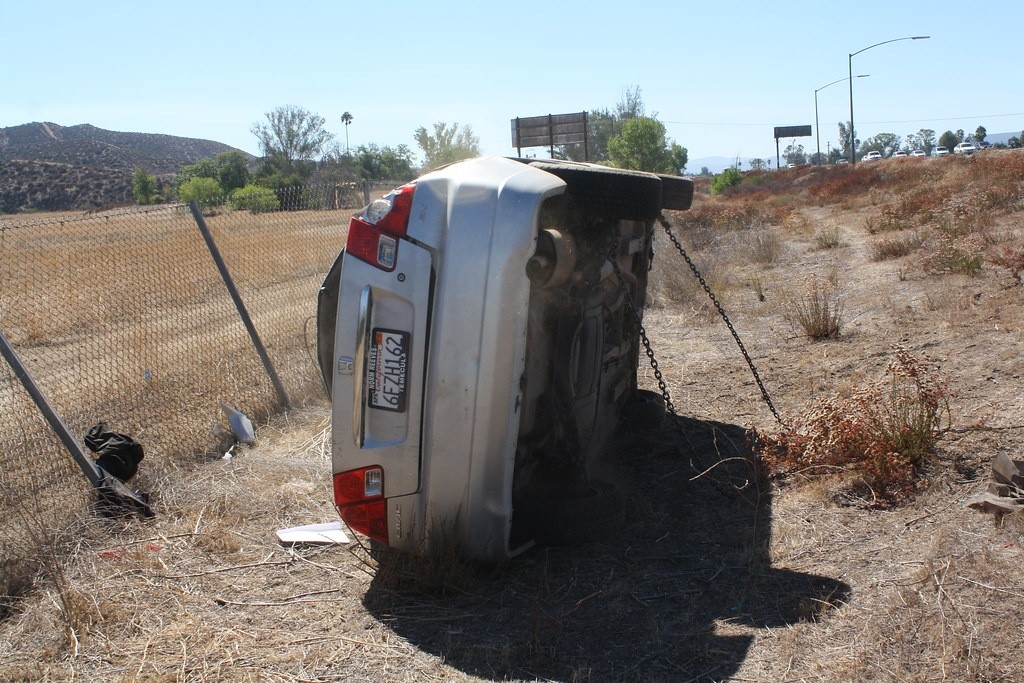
[860,151,882,162]
[314,154,694,566]
[892,151,907,158]
[954,143,976,155]
[930,146,950,157]
[787,164,795,168]
[910,150,927,158]
[835,159,848,165]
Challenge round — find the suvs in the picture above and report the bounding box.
[974,141,993,150]
